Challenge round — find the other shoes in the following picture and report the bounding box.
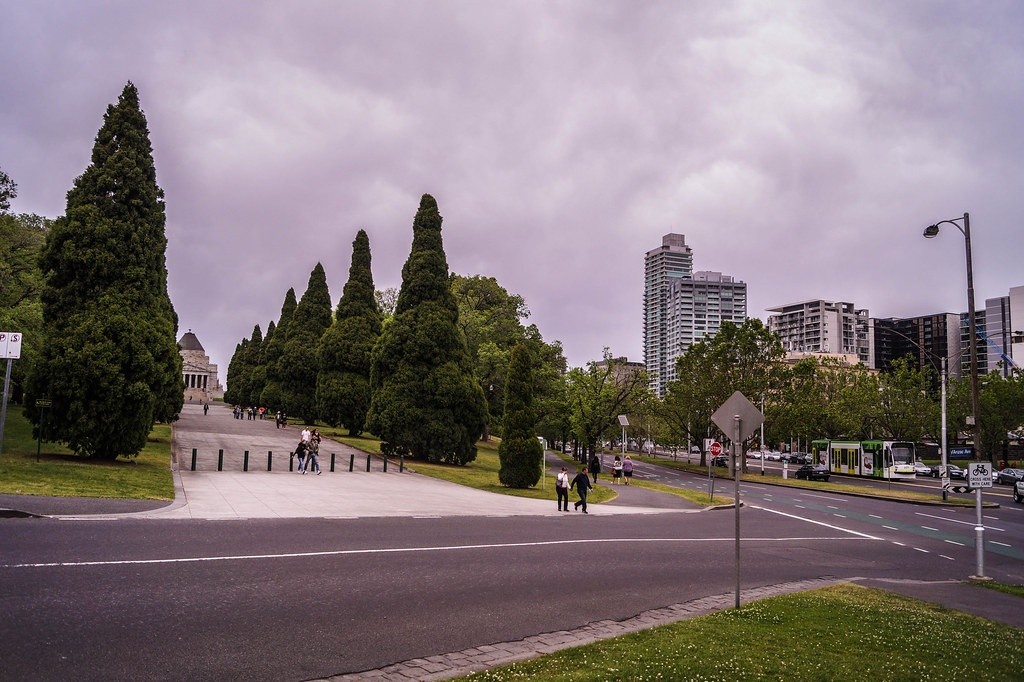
[610,482,614,485]
[558,509,561,511]
[305,471,308,474]
[564,510,569,511]
[582,510,588,513]
[317,471,321,475]
[297,471,300,473]
[624,481,628,485]
[303,471,305,474]
[575,503,577,510]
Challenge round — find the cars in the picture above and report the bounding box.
[962,465,999,483]
[554,440,590,464]
[915,461,932,476]
[1013,474,1024,503]
[710,456,729,468]
[997,467,1024,485]
[689,445,813,465]
[929,463,967,480]
[666,446,678,452]
[794,464,831,482]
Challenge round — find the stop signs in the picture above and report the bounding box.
[710,442,722,456]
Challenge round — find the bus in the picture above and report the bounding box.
[811,438,918,482]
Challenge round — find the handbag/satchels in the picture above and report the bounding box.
[555,476,563,487]
[611,467,616,474]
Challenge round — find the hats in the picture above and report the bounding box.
[277,411,280,413]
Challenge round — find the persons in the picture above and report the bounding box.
[622,456,633,485]
[204,403,208,415]
[311,428,322,456]
[200,398,202,404]
[556,467,572,511]
[570,467,593,513]
[233,405,268,421]
[611,456,623,485]
[190,395,192,400]
[282,413,287,428]
[291,439,306,475]
[275,410,282,429]
[301,426,310,443]
[304,435,322,475]
[590,456,600,483]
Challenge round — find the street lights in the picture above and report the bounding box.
[862,324,1024,495]
